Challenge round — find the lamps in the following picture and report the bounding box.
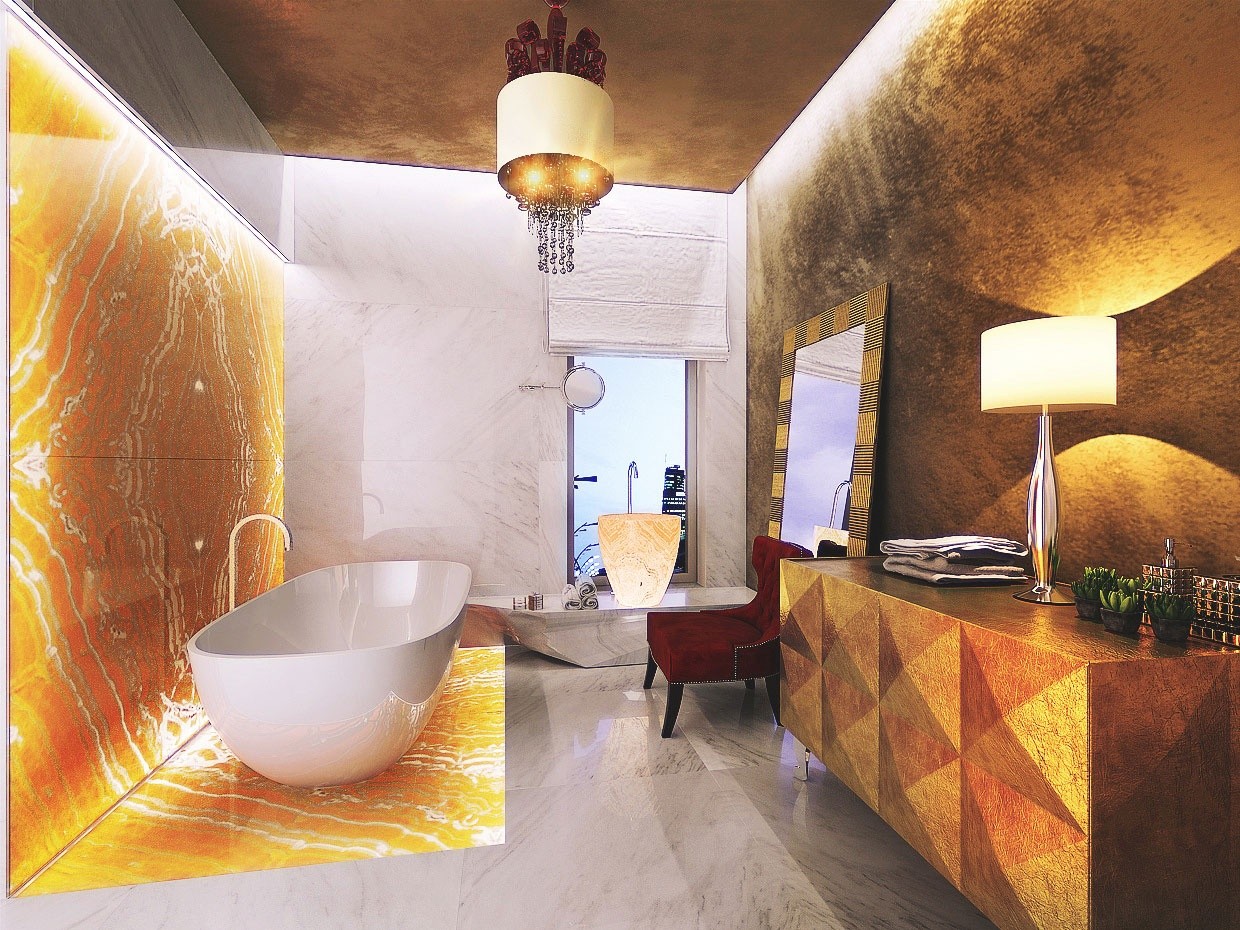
[494,0,615,275]
[981,313,1120,606]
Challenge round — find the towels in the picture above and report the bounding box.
[885,551,1029,585]
[880,536,1029,559]
[561,584,582,611]
[581,595,600,609]
[575,574,597,596]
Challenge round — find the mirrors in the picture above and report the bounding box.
[768,282,891,559]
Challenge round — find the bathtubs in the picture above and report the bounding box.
[361,525,487,569]
[185,560,476,790]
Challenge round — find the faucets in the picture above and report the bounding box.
[363,494,384,517]
[227,513,292,608]
[830,480,853,527]
[101,514,169,698]
[627,460,638,513]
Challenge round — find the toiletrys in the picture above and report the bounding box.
[1141,539,1198,616]
[1192,571,1240,627]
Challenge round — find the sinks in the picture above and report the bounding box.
[812,526,846,556]
[598,513,681,608]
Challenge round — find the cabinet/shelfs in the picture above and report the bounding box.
[777,557,1240,930]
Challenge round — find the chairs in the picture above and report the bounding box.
[642,534,813,740]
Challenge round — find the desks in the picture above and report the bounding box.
[468,585,759,670]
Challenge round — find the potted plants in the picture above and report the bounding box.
[1099,588,1148,633]
[1080,566,1119,595]
[1142,591,1197,643]
[1071,575,1107,621]
